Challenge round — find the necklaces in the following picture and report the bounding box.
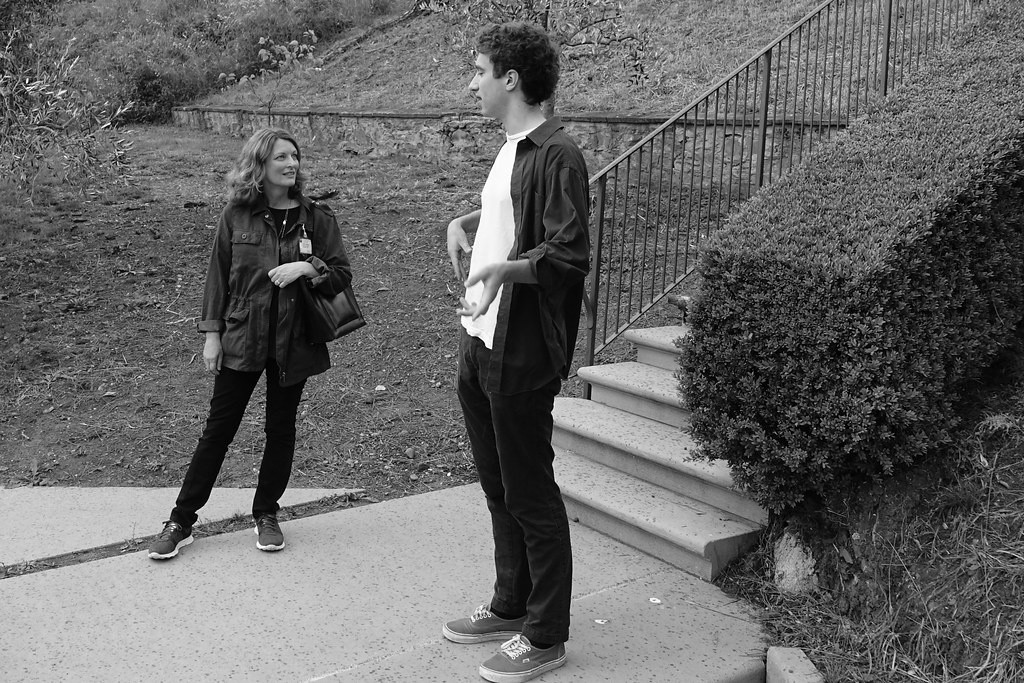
[277,198,289,237]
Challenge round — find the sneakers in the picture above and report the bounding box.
[479,634,567,683]
[254,512,286,551]
[441,603,527,644]
[148,520,194,560]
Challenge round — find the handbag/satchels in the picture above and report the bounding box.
[305,282,366,339]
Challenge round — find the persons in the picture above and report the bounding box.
[443,21,589,683]
[145,126,352,559]
